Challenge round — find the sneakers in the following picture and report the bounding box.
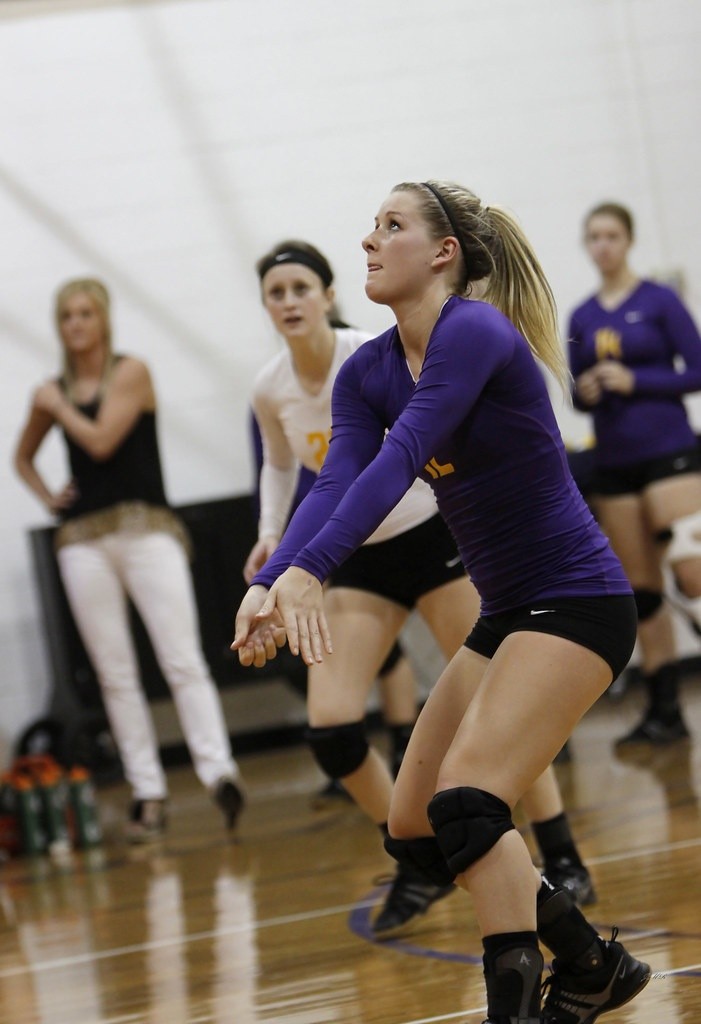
[211,778,245,831]
[538,924,652,1024]
[308,780,356,811]
[477,1012,550,1023]
[542,864,599,907]
[107,813,167,843]
[552,741,571,764]
[614,700,690,749]
[370,863,459,937]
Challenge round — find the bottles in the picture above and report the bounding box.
[1,759,101,861]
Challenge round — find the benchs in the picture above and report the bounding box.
[30,495,307,790]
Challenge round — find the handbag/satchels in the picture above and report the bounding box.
[1,713,104,860]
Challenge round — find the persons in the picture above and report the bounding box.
[243,241,600,941]
[566,203,701,748]
[13,279,250,845]
[229,179,652,1024]
